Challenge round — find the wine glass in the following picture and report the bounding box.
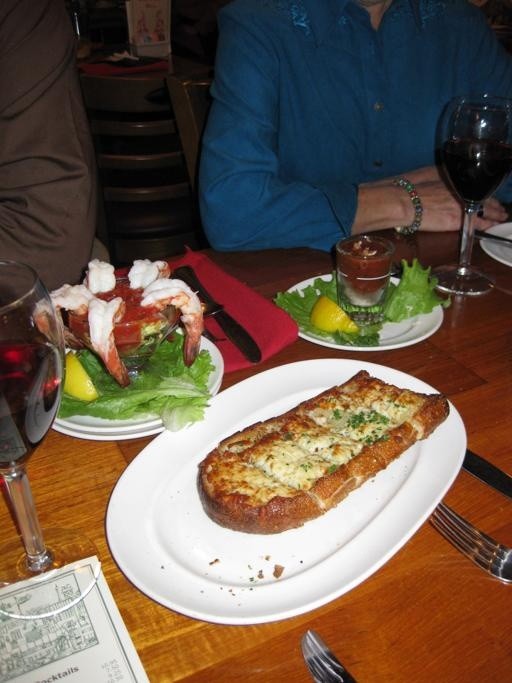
[67,278,181,398]
[1,260,100,618]
[428,91,511,299]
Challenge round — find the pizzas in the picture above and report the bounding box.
[198,369,450,535]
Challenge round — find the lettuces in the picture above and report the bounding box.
[56,331,216,430]
[274,259,453,348]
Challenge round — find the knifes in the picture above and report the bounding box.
[467,449,512,502]
[171,266,265,364]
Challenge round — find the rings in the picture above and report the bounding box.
[477,205,484,218]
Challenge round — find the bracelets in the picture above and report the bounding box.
[390,176,421,233]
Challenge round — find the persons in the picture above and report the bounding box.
[0,0,101,300]
[196,0,510,253]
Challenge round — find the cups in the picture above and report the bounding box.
[331,235,396,323]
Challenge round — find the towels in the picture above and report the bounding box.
[114,247,298,375]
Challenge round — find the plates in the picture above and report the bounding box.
[275,272,445,352]
[478,222,512,267]
[50,328,222,442]
[105,358,466,625]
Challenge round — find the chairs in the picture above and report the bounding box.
[78,74,193,266]
[166,74,212,190]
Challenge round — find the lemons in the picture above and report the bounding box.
[310,295,358,334]
[62,349,99,402]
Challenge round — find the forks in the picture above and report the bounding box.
[429,503,510,592]
[295,627,357,682]
